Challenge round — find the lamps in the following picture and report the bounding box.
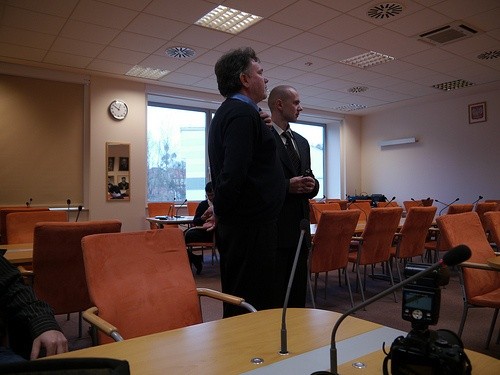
[380,137,417,146]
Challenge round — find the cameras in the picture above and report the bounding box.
[390,287,471,375]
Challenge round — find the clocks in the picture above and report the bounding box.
[108,101,128,121]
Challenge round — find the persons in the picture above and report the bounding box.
[267,86,319,308]
[207,48,283,320]
[184,182,214,274]
[109,185,123,199]
[0,254,69,362]
[108,177,113,192]
[119,177,128,189]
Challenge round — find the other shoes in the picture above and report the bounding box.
[194,255,203,274]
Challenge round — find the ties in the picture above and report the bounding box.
[282,131,300,176]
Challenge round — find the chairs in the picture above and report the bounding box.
[0,200,500,353]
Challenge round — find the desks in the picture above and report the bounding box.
[310,215,439,236]
[145,214,194,229]
[36,308,500,375]
[0,243,34,265]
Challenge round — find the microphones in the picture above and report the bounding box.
[67,198,82,223]
[311,244,472,375]
[26,198,33,207]
[171,197,187,219]
[278,220,308,355]
[319,193,483,217]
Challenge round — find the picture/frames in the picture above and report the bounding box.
[468,101,487,125]
